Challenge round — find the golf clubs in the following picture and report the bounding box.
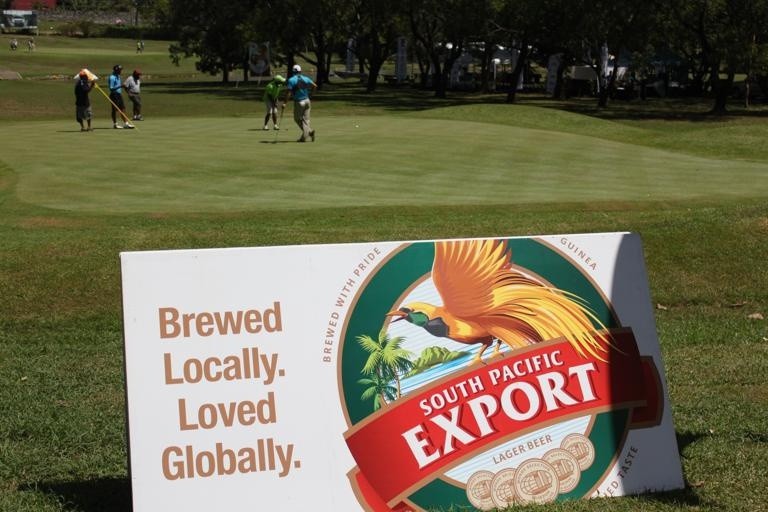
[271,108,284,143]
[279,106,288,131]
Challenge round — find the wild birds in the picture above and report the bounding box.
[384,239,629,368]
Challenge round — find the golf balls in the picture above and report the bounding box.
[355,125,359,128]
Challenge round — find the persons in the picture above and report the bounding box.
[10,38,18,50]
[264,75,285,129]
[125,66,147,121]
[140,41,145,52]
[27,36,33,55]
[282,64,318,142]
[402,56,657,93]
[108,64,134,128]
[74,71,95,131]
[136,39,141,54]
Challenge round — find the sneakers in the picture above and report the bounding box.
[125,124,135,129]
[297,138,305,142]
[114,125,124,129]
[274,126,279,130]
[263,126,270,130]
[310,129,315,141]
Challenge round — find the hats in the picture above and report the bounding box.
[135,68,142,74]
[293,65,301,72]
[274,75,286,83]
